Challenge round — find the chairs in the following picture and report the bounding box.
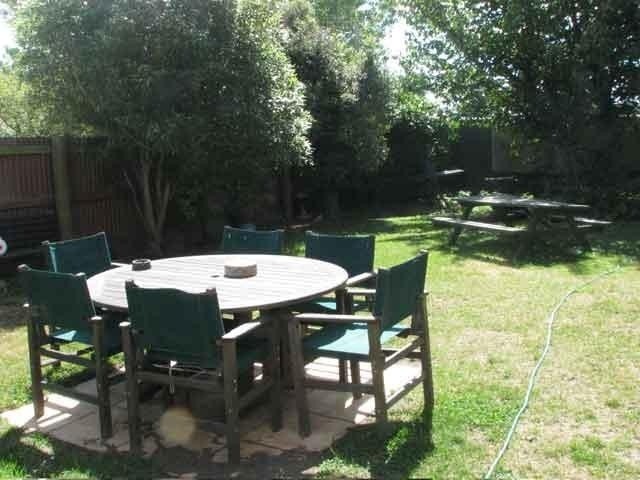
[221,226,284,256]
[286,249,433,441]
[17,264,123,439]
[41,230,128,282]
[119,279,283,465]
[294,229,376,385]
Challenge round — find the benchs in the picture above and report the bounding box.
[553,215,613,252]
[431,217,546,266]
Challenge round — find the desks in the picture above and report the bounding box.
[87,254,348,422]
[453,196,591,263]
